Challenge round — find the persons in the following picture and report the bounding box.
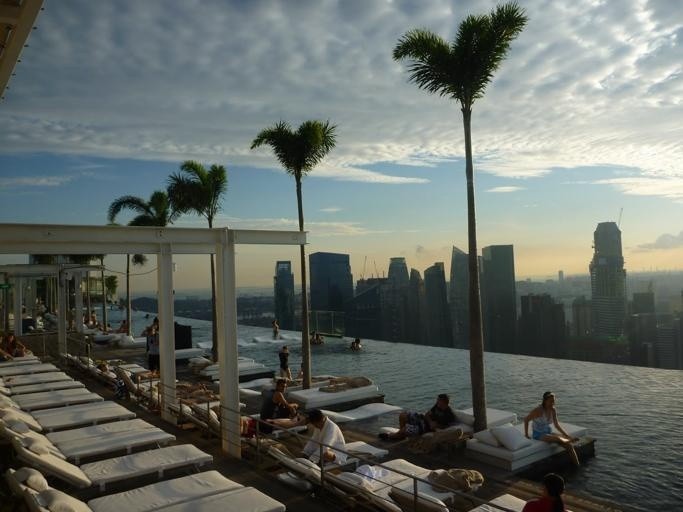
[521,473,572,512]
[260,379,299,421]
[524,390,581,465]
[271,320,362,384]
[222,412,300,435]
[392,393,452,439]
[302,410,347,466]
[0,303,160,382]
[172,380,219,405]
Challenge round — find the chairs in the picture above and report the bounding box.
[0,349,528,511]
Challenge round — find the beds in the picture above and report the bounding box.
[464,418,587,470]
[67,320,302,348]
